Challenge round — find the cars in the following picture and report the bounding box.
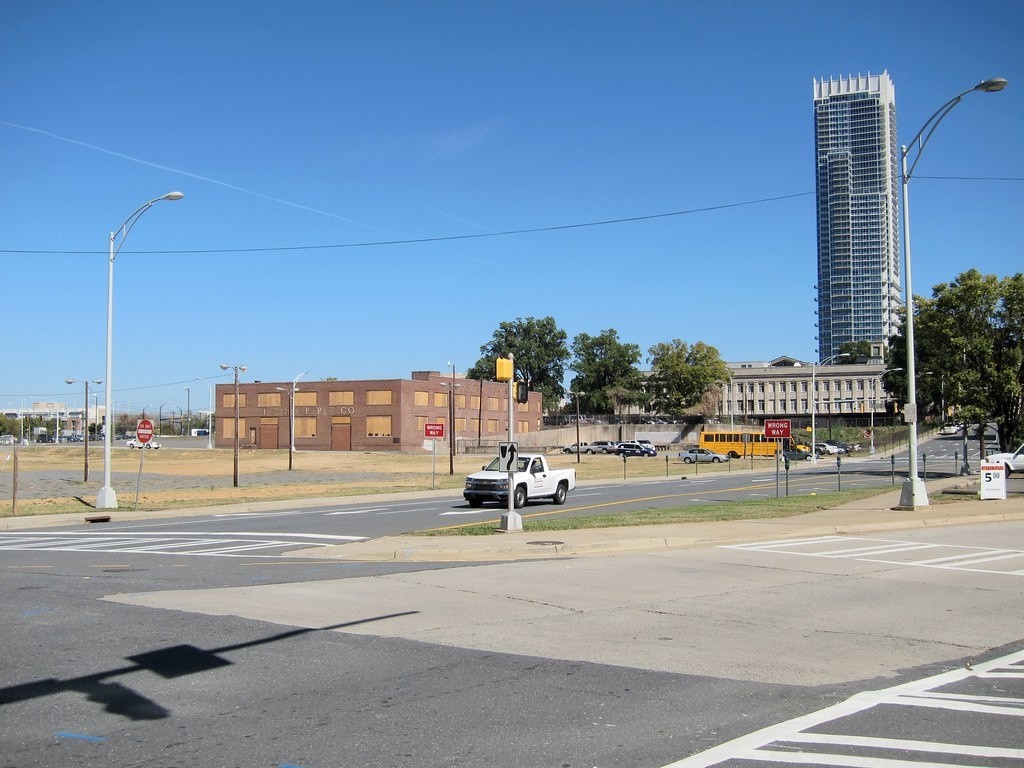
[774,438,862,462]
[562,441,658,457]
[677,448,731,464]
[940,421,957,434]
[0,430,137,445]
[982,440,1024,477]
[125,438,162,450]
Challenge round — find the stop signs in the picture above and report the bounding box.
[136,419,155,443]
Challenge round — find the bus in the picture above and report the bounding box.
[697,430,811,459]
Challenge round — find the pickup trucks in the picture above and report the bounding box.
[463,453,577,509]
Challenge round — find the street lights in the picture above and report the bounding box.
[440,382,462,476]
[289,371,310,453]
[566,390,586,464]
[219,364,248,488]
[807,352,851,465]
[185,386,191,437]
[891,76,1013,510]
[93,393,98,444]
[65,376,103,482]
[195,377,214,449]
[276,386,300,472]
[93,189,186,510]
[869,367,903,458]
